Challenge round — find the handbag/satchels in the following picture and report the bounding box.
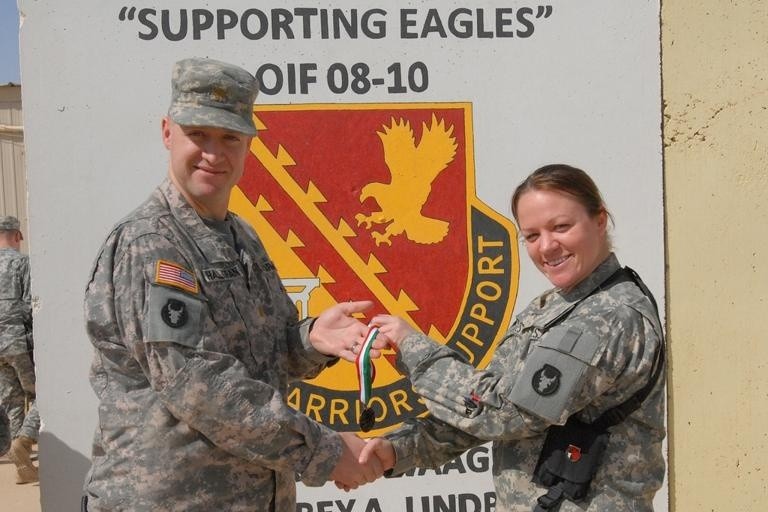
[529,420,607,509]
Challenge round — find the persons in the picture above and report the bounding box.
[0,215,40,484]
[82,58,380,512]
[336,165,664,511]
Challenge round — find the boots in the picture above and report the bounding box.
[9,430,38,485]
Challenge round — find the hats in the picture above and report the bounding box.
[0,215,25,240]
[168,56,259,137]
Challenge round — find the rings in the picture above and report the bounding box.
[351,344,357,350]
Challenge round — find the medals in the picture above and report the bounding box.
[360,408,375,432]
